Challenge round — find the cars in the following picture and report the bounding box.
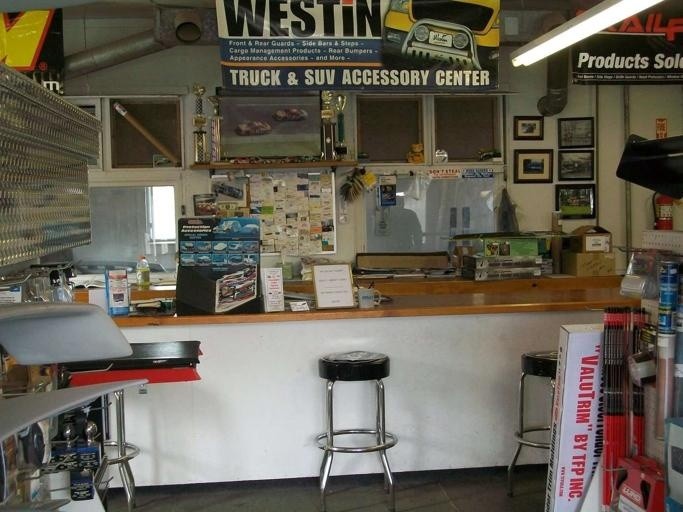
[195,180,245,215]
[380,0,496,78]
[179,218,260,267]
[523,161,541,172]
[235,119,272,135]
[521,125,534,132]
[556,126,590,206]
[273,108,309,123]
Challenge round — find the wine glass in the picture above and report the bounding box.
[28,266,77,305]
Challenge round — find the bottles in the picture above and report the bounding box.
[134,256,150,291]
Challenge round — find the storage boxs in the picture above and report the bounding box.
[569,225,613,251]
[561,249,616,277]
[545,323,603,511]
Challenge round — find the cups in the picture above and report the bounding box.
[358,287,382,310]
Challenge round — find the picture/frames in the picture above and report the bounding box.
[514,148,554,183]
[514,114,542,142]
[555,185,597,219]
[558,116,596,148]
[561,149,595,183]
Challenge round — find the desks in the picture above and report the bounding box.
[100,274,641,491]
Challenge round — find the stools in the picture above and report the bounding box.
[506,351,557,497]
[101,384,140,511]
[315,351,398,510]
